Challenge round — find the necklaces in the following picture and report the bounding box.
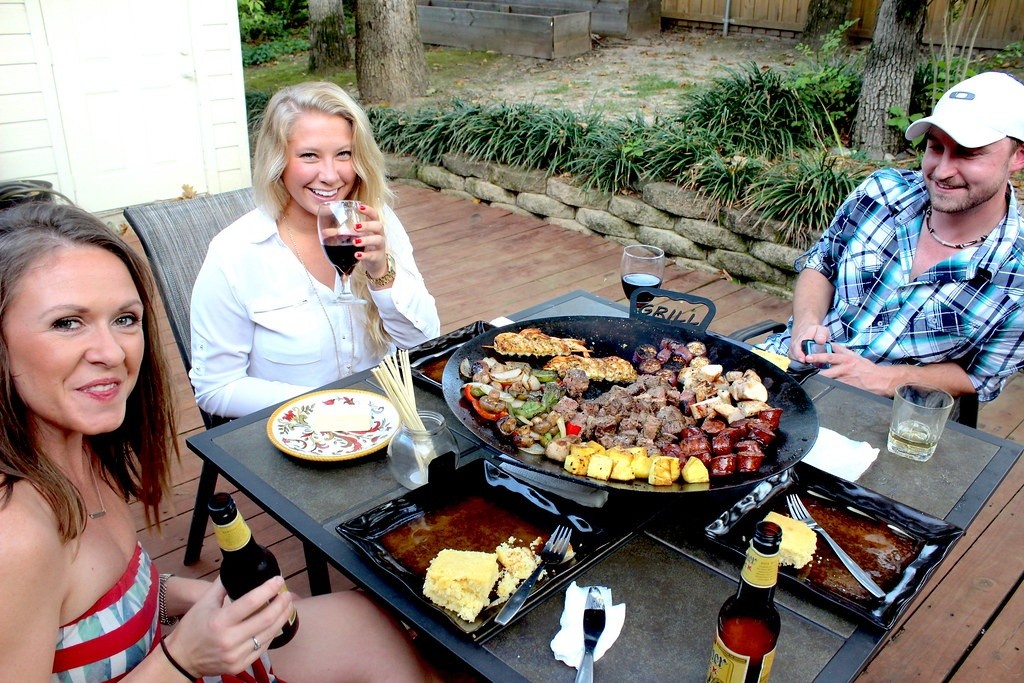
[83,445,106,518]
[926,205,989,249]
[284,214,355,380]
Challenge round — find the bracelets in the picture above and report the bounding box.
[159,637,198,683]
[158,573,178,626]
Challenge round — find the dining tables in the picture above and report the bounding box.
[186,290,1023,683]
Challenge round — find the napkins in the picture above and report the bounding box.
[801,426,880,482]
[551,582,626,670]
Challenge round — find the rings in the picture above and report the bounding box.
[252,636,261,649]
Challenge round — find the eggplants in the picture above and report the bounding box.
[631,338,707,388]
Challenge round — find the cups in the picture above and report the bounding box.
[886,381,953,462]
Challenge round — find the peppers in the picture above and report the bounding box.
[465,369,581,445]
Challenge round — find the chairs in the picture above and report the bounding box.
[121,187,329,596]
[727,314,979,429]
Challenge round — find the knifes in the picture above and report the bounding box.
[576,589,607,683]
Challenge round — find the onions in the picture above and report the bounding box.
[460,357,567,454]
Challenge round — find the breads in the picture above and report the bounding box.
[764,511,817,570]
[423,542,575,623]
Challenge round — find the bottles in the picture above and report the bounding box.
[387,410,461,488]
[207,492,298,651]
[706,521,783,683]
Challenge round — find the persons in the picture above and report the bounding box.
[188,81,440,416]
[752,72,1024,422]
[0,201,443,683]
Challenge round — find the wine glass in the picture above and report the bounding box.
[315,202,369,305]
[621,245,664,316]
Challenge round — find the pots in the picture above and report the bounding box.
[441,315,819,496]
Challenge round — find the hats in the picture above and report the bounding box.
[904,71,1024,149]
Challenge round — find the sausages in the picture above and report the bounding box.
[660,408,782,478]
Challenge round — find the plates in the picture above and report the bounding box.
[703,463,971,628]
[267,388,402,461]
[392,321,502,393]
[333,458,647,648]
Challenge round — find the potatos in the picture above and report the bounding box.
[564,441,710,486]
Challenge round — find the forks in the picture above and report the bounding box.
[786,491,886,600]
[494,524,574,625]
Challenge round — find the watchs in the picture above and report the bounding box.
[365,253,396,287]
